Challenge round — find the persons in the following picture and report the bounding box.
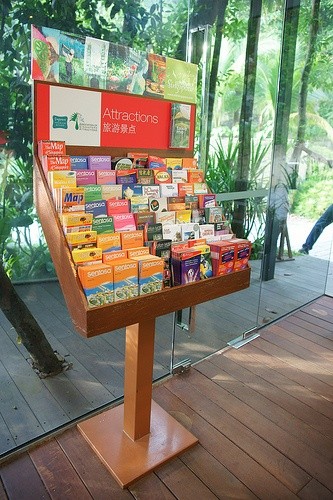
[298,144,333,256]
[61,43,75,84]
[128,58,150,95]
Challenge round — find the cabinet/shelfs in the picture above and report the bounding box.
[31,78,250,490]
